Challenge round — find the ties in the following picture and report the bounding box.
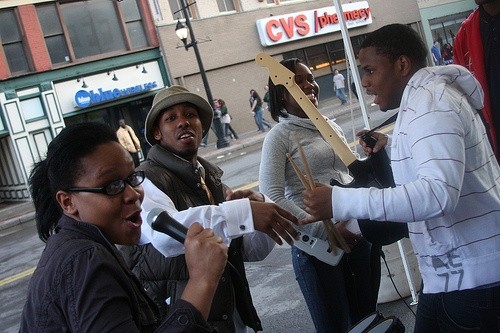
[200,173,215,205]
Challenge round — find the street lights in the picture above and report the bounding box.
[174,0,232,149]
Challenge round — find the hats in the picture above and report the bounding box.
[145,86,214,147]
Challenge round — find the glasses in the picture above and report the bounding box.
[66,170,146,197]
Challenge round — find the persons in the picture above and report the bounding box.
[441,43,454,65]
[263,84,279,123]
[449,30,455,43]
[249,90,271,132]
[435,33,443,46]
[333,69,348,105]
[446,43,454,62]
[212,99,233,139]
[347,63,359,102]
[431,40,443,66]
[18,121,229,332]
[116,118,141,169]
[217,99,239,142]
[452,0,500,167]
[198,132,209,148]
[251,91,272,134]
[297,22,499,333]
[114,85,302,333]
[257,57,384,333]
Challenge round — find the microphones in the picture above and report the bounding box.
[147,207,188,245]
